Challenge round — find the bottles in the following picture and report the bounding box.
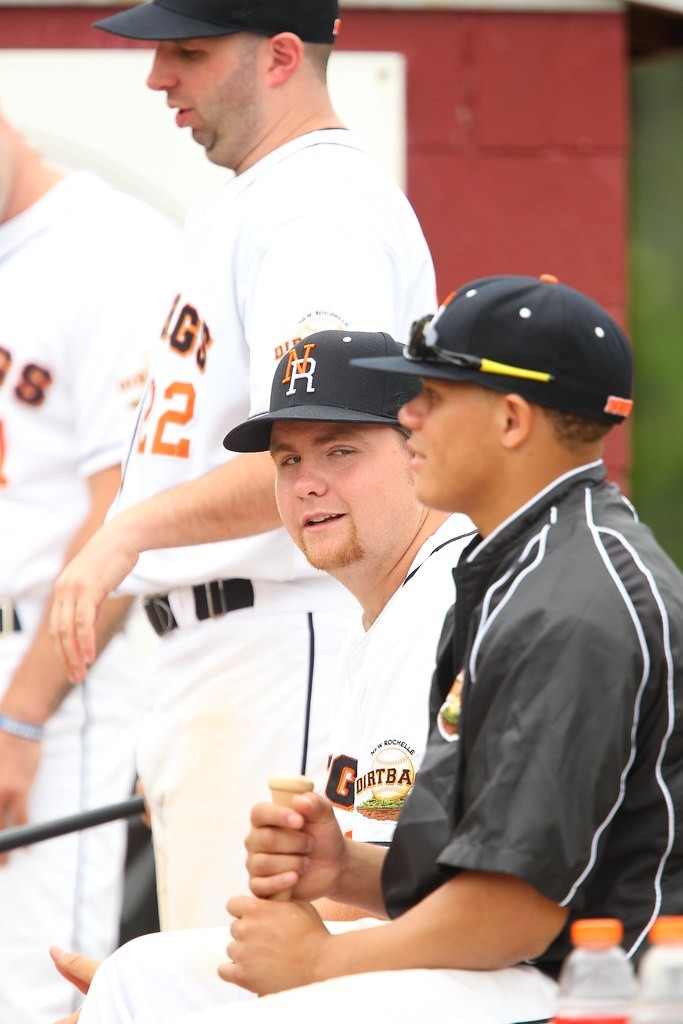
[631,916,683,1024]
[555,919,639,1024]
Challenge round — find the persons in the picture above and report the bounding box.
[0,1,474,1024]
[75,273,683,1024]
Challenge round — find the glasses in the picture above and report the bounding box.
[402,314,557,383]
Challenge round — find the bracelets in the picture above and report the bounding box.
[0,717,45,741]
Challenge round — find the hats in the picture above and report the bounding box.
[350,274,633,425]
[92,0,339,44]
[223,330,423,453]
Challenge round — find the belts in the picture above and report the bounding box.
[144,578,254,637]
[0,610,21,631]
